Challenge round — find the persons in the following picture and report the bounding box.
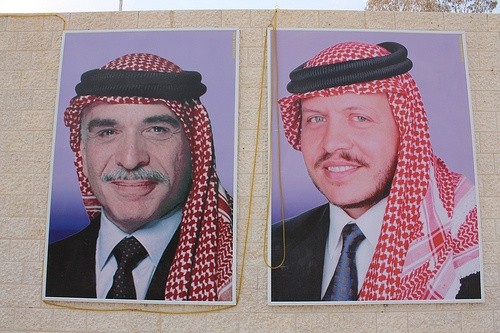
[271,39,482,302]
[45,53,233,302]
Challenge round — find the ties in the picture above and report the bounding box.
[105,236,148,299]
[322,222,365,301]
